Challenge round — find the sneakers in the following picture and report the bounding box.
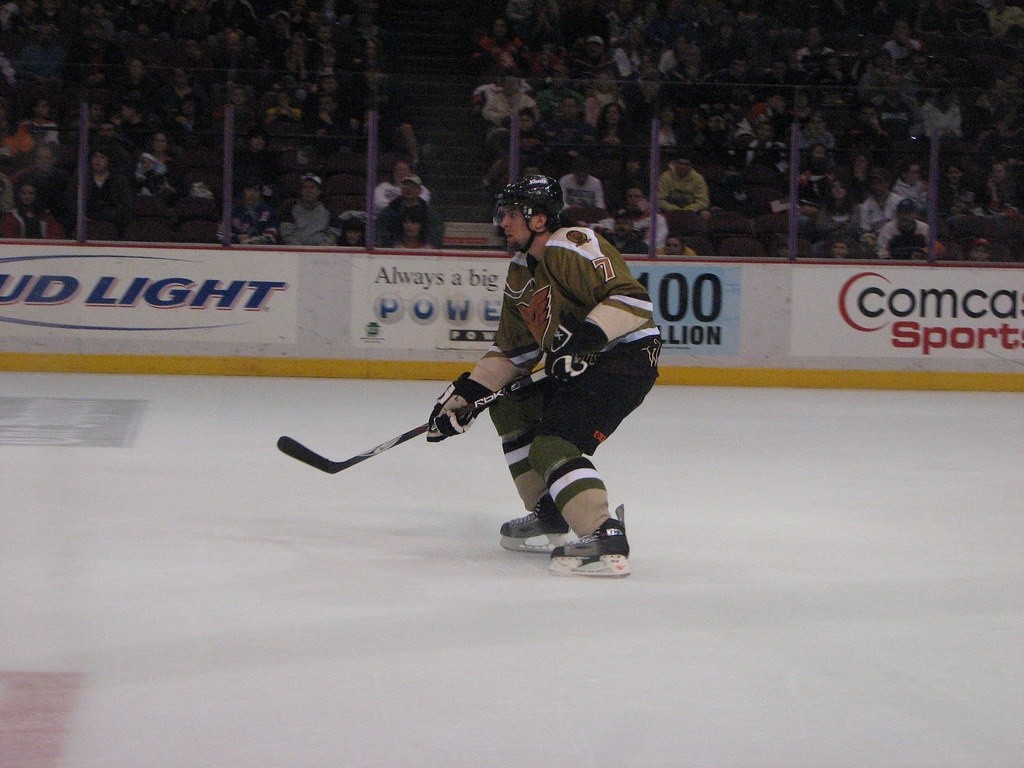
[500,494,578,553]
[547,504,630,575]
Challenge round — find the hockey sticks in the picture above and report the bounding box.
[277,368,550,476]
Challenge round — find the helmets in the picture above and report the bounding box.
[495,175,564,220]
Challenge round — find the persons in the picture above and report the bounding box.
[0,0,1024,263]
[427,176,662,560]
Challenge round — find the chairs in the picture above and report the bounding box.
[475,25,1023,260]
[51,94,367,250]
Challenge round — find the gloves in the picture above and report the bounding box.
[427,372,497,443]
[542,318,606,392]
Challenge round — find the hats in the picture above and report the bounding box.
[897,199,915,211]
[402,172,423,186]
[301,172,322,184]
